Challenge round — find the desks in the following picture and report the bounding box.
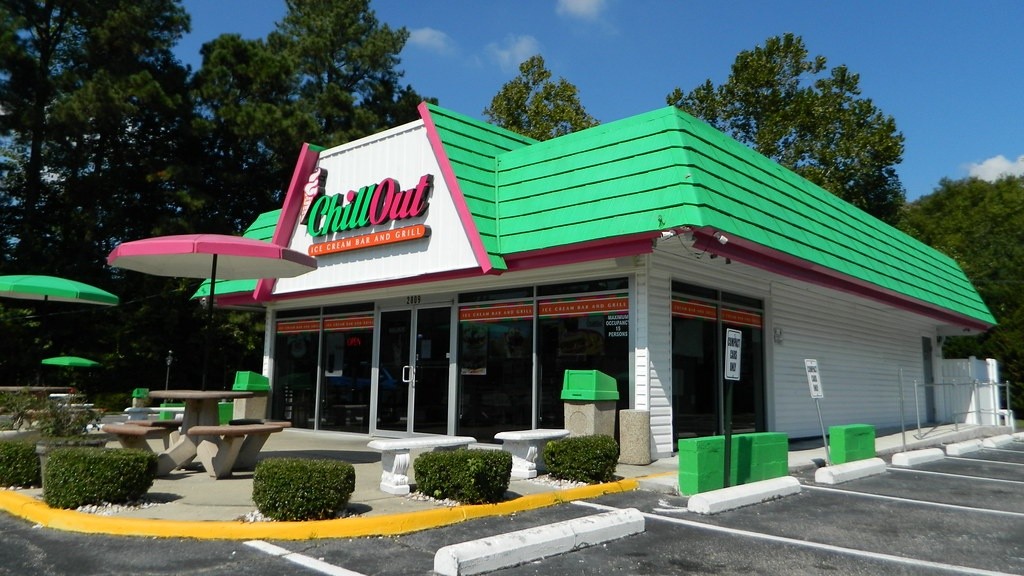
[50,394,85,403]
[149,389,254,479]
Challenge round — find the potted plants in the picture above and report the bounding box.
[0,386,108,499]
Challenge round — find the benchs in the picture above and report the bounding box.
[366,436,477,495]
[124,406,185,420]
[55,404,94,407]
[102,419,293,479]
[494,429,571,480]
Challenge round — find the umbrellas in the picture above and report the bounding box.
[0,273,119,400]
[105,233,317,394]
[40,355,103,386]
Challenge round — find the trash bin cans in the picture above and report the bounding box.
[232,370,270,422]
[561,370,620,439]
[131,388,149,407]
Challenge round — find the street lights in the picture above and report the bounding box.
[164,349,175,390]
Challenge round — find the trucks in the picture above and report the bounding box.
[324,360,405,423]
